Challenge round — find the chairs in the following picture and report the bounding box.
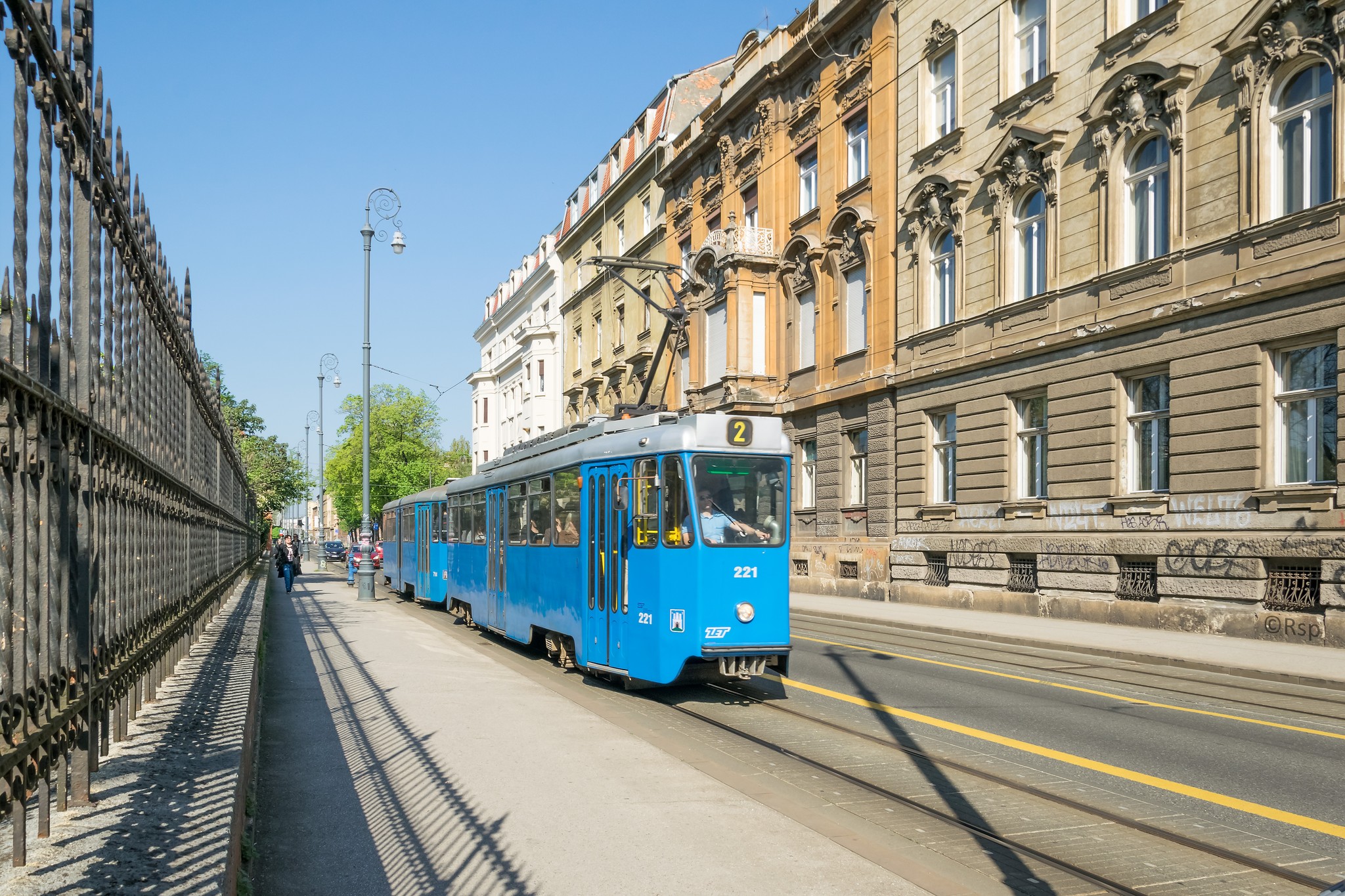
[352,547,360,552]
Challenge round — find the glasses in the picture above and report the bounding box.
[293,536,297,537]
[285,539,291,540]
[699,495,711,501]
[533,517,542,521]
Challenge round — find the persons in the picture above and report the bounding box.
[544,512,579,544]
[275,535,302,594]
[273,533,303,572]
[434,505,449,542]
[680,487,771,546]
[520,510,546,544]
[708,474,735,515]
[466,516,486,542]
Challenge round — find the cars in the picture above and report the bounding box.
[374,540,383,560]
[325,539,348,562]
[345,541,380,569]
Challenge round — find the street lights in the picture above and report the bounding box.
[315,353,342,572]
[296,440,309,539]
[303,409,320,561]
[356,186,407,602]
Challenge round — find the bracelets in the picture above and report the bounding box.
[754,529,760,535]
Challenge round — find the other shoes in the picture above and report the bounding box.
[290,588,292,593]
[286,591,290,594]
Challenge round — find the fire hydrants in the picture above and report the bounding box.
[346,550,357,588]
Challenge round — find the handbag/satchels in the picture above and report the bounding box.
[293,558,298,566]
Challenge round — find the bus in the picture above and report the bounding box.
[444,255,792,691]
[381,477,467,606]
